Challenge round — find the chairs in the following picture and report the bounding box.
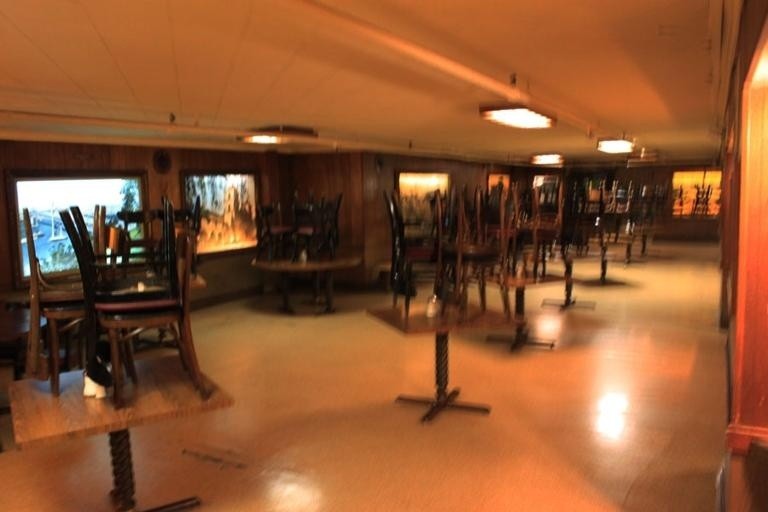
[672,184,713,216]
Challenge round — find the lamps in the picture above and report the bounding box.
[478,104,556,128]
[596,139,636,154]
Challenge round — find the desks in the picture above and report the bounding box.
[0,222,650,512]
